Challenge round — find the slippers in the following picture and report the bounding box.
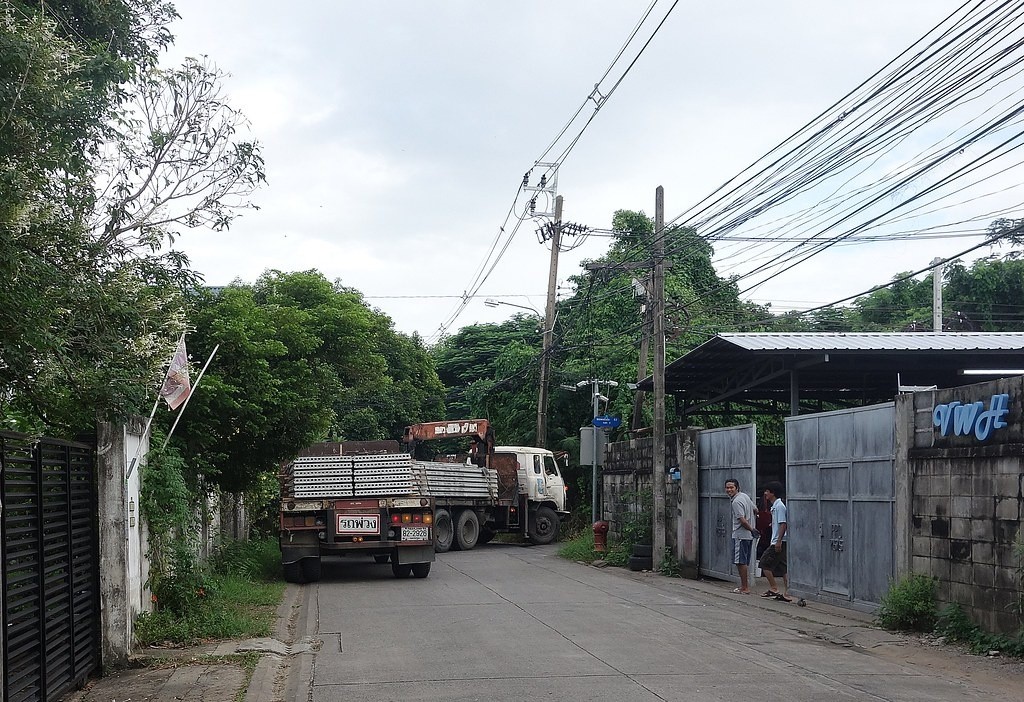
[761,589,779,598]
[730,587,751,595]
[773,594,792,603]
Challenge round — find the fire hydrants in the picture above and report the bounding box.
[592,520,610,552]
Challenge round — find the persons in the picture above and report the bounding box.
[724,479,761,594]
[757,482,793,601]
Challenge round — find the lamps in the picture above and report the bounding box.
[957,367,1024,375]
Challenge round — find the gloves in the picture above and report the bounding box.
[751,528,760,539]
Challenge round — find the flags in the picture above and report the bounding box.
[162,337,191,410]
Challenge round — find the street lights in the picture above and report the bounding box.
[484,297,542,328]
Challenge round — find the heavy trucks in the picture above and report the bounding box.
[272,444,567,583]
